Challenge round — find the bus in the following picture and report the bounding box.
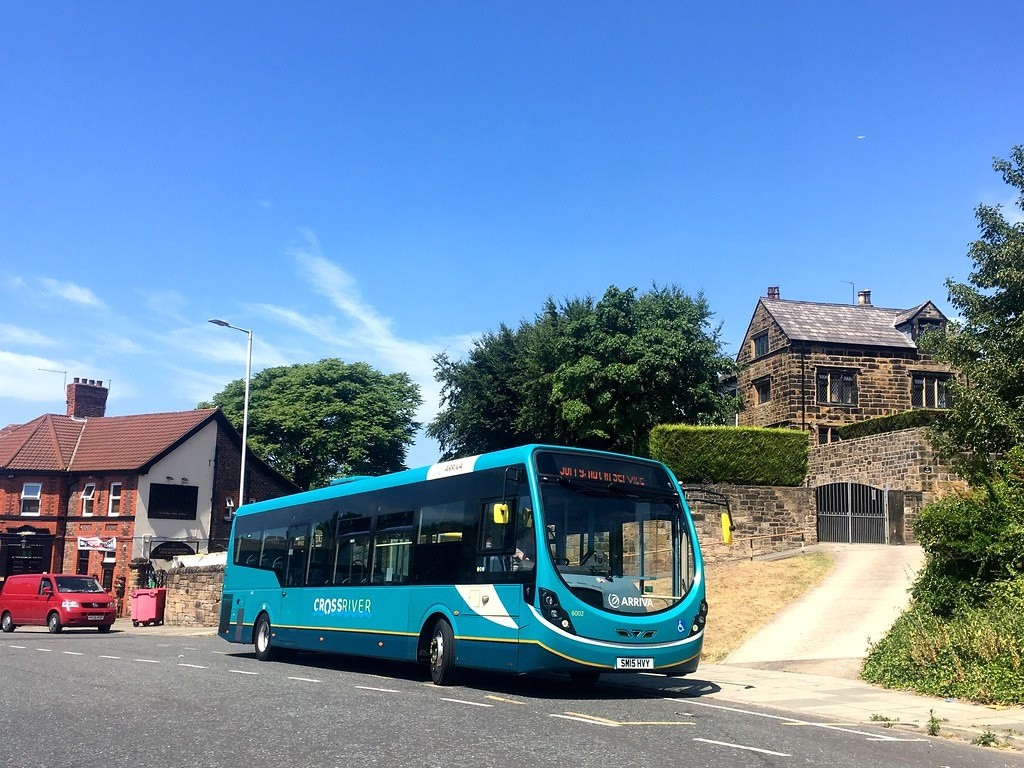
[218,442,737,688]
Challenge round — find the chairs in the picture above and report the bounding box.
[246,553,376,586]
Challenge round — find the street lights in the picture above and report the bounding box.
[207,319,253,507]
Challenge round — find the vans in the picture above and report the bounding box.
[0,573,118,634]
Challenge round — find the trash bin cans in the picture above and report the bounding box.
[130,588,167,627]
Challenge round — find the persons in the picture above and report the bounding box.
[510,528,535,572]
[314,552,343,583]
[92,573,99,590]
